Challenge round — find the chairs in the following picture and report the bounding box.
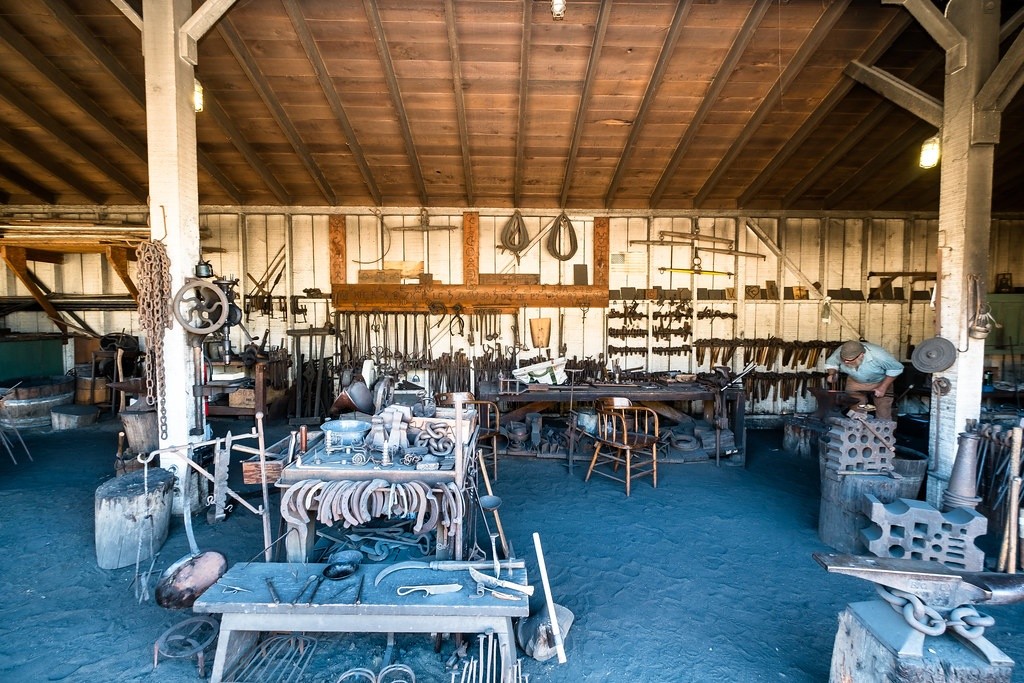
[434,392,500,480]
[585,397,659,497]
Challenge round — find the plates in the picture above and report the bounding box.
[322,561,360,581]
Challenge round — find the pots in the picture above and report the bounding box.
[807,386,895,411]
[154,443,228,609]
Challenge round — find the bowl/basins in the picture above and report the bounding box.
[478,495,503,512]
[320,419,371,445]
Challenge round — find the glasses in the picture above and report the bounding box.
[842,353,861,361]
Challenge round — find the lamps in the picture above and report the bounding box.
[195,80,204,113]
[919,135,941,169]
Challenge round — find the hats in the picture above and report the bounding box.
[841,341,867,358]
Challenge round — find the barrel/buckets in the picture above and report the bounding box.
[818,436,929,555]
[617,415,634,432]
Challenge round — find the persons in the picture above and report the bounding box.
[824,341,904,421]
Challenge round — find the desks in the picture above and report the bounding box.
[276,408,477,563]
[477,379,719,469]
[191,561,529,683]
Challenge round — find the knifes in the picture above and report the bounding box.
[469,567,534,596]
